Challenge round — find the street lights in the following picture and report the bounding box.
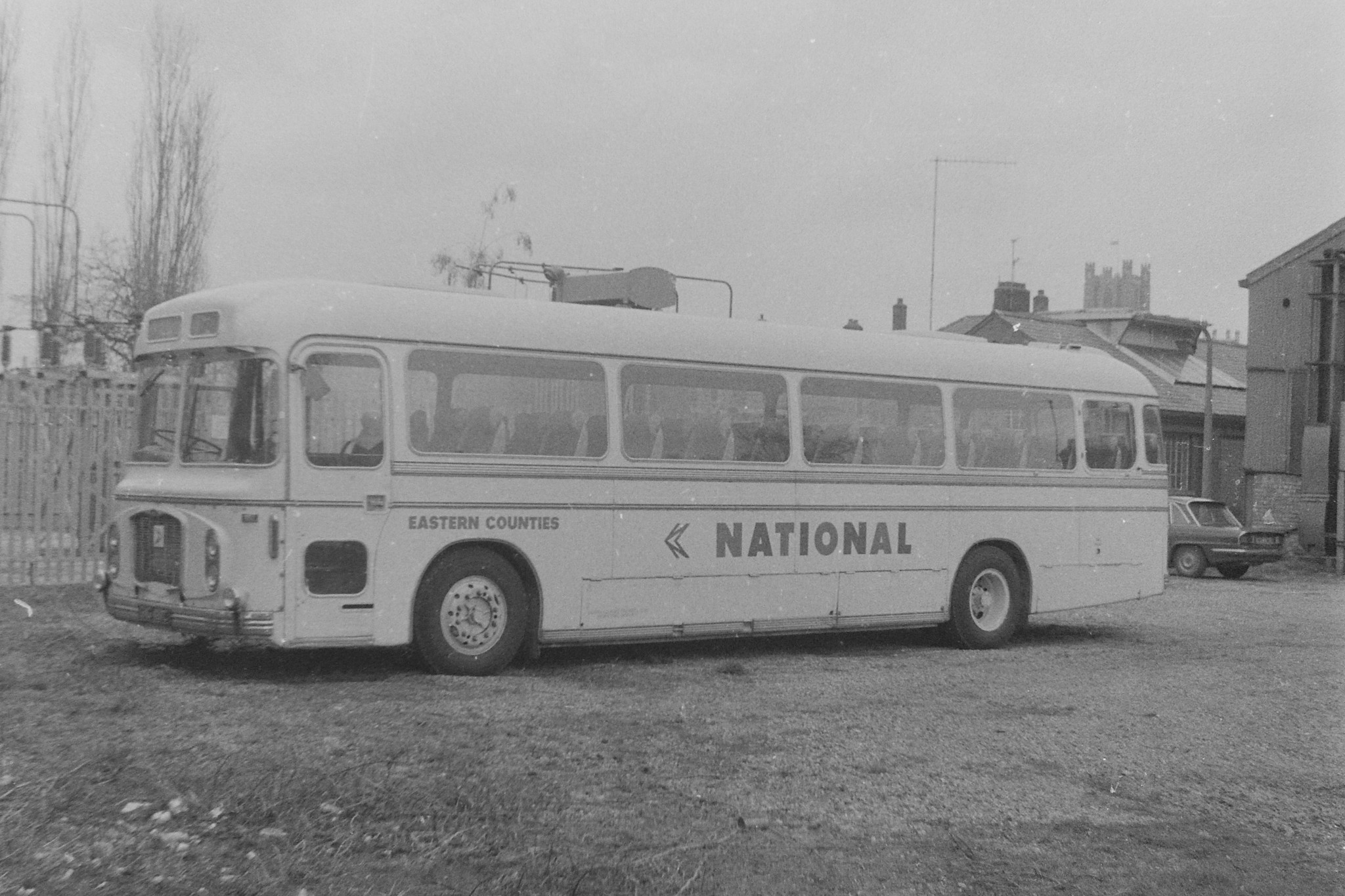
[1178,322,1216,498]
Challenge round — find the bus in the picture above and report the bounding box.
[92,268,1173,673]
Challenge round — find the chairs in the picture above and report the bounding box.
[351,404,1159,470]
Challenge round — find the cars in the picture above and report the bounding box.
[1169,492,1290,579]
